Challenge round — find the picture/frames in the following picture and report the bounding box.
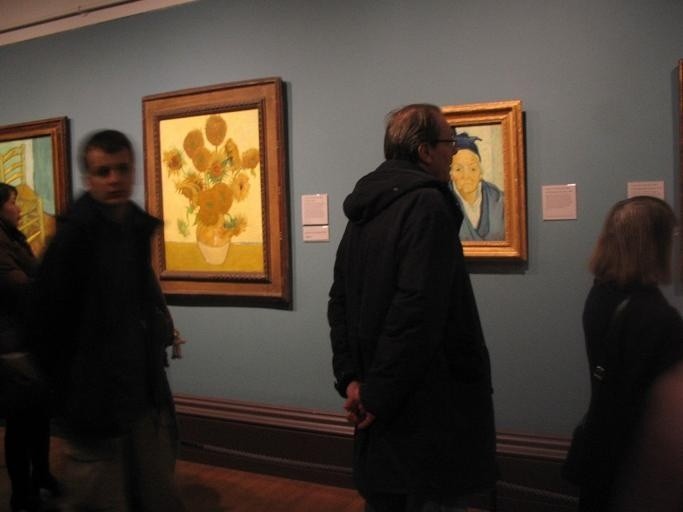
[0,116,73,256]
[438,98,527,265]
[140,76,294,309]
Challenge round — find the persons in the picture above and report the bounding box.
[563,195,683,512]
[449,133,505,242]
[327,103,499,512]
[18,130,185,512]
[609,358,683,512]
[0,183,68,512]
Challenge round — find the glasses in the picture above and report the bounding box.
[438,138,457,146]
